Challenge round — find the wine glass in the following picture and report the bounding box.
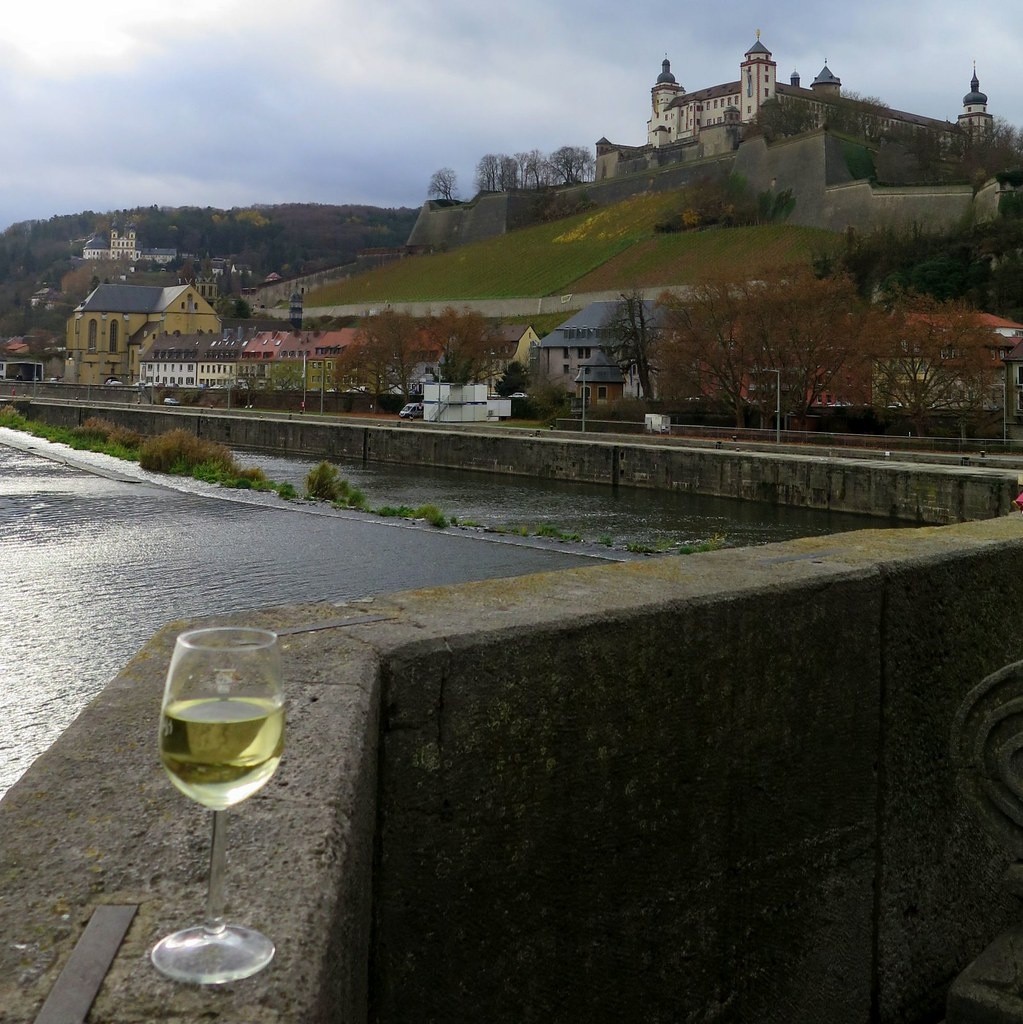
[150,627,290,982]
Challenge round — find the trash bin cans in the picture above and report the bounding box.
[644,414,671,435]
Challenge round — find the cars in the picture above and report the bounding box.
[209,384,226,389]
[163,397,181,406]
[132,381,178,387]
[105,379,123,386]
[508,392,529,398]
[487,391,500,398]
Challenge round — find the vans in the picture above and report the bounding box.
[399,402,424,418]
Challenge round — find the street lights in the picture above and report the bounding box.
[309,361,325,415]
[569,367,586,431]
[762,368,781,444]
